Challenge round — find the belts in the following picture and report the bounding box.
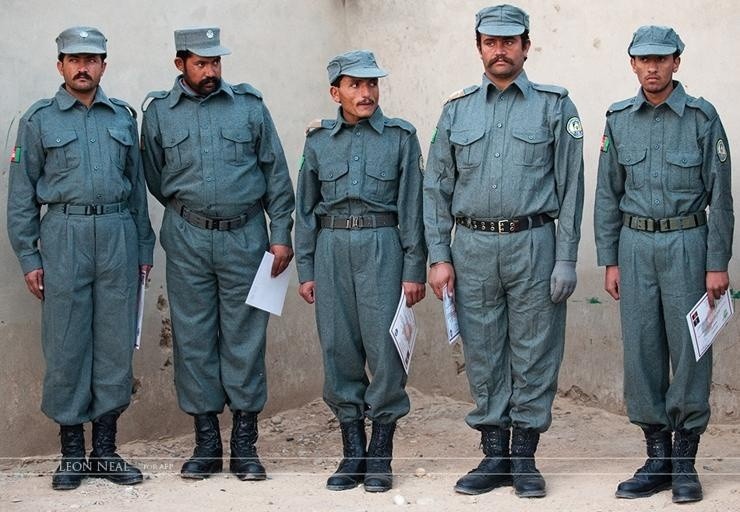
[456,214,553,234]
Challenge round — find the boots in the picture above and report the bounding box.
[54,415,704,501]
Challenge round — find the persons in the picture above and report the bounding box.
[7,26,156,490]
[141,26,295,481]
[593,27,734,503]
[295,50,428,492]
[422,6,584,497]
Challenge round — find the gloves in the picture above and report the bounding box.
[550,260,577,303]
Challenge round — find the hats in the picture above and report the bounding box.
[327,49,388,83]
[628,25,685,56]
[476,5,529,36]
[174,27,232,57]
[56,26,107,53]
